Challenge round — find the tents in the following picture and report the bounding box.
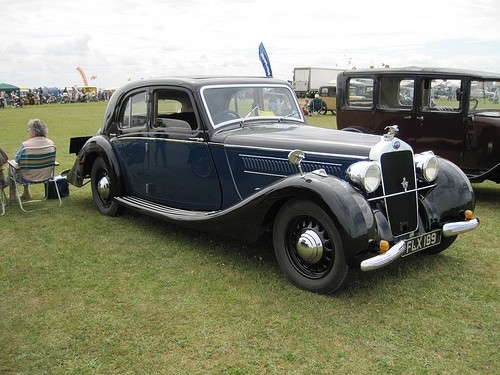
[329,79,500,98]
[0,83,21,105]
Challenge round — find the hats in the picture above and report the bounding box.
[315,94,319,98]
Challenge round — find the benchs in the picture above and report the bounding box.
[133,109,230,131]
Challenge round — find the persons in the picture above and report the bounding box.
[435,86,455,102]
[5,119,54,201]
[237,88,321,116]
[0,87,120,109]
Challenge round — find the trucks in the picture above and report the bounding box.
[293,67,347,98]
[64,86,97,103]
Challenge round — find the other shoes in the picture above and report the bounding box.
[8,196,19,203]
[20,195,31,200]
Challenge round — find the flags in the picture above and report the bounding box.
[259,42,272,76]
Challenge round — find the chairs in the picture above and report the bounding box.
[0,145,62,216]
[306,98,323,116]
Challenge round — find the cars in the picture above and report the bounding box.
[315,85,367,115]
[61,73,480,297]
[335,65,500,186]
[354,78,500,108]
